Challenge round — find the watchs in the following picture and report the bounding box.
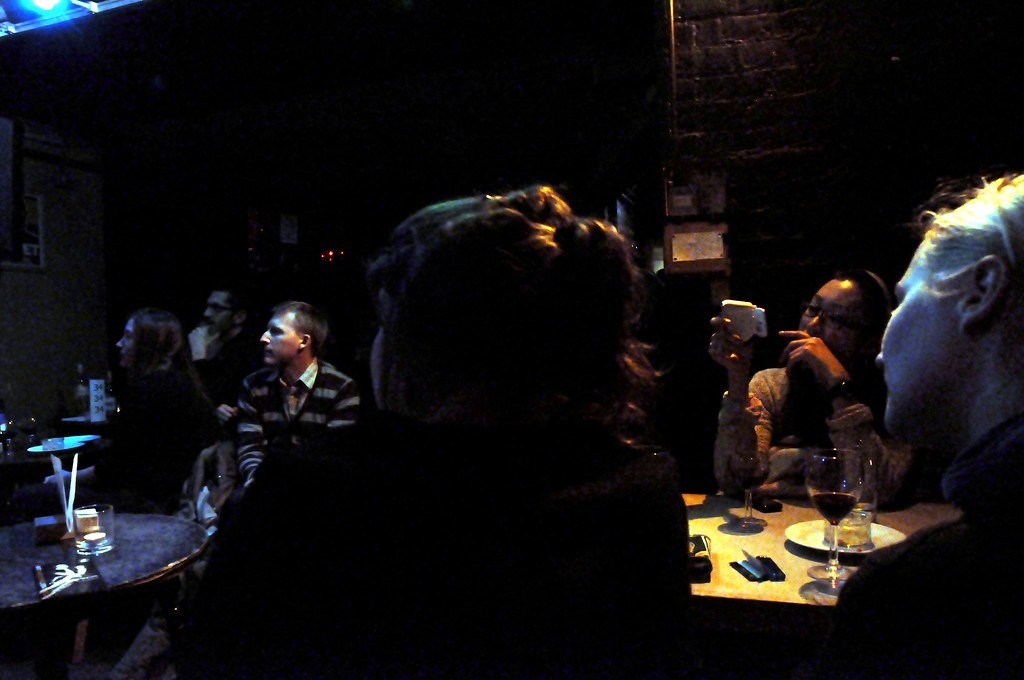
[821,379,857,407]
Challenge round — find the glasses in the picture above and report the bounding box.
[800,301,859,330]
[206,303,234,312]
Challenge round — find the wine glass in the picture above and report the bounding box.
[727,446,772,528]
[805,448,863,581]
[18,417,36,449]
[0,414,19,457]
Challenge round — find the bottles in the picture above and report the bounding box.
[75,364,90,414]
[106,371,118,420]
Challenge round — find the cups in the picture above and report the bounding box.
[41,438,64,451]
[826,503,875,548]
[72,504,116,556]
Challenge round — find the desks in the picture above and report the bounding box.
[0,513,210,680]
[0,444,95,491]
[681,494,965,608]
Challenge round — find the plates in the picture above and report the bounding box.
[784,520,904,554]
[43,434,100,443]
[27,442,87,454]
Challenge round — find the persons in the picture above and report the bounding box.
[702,265,922,512]
[808,171,1024,679]
[44,285,361,680]
[178,183,693,680]
